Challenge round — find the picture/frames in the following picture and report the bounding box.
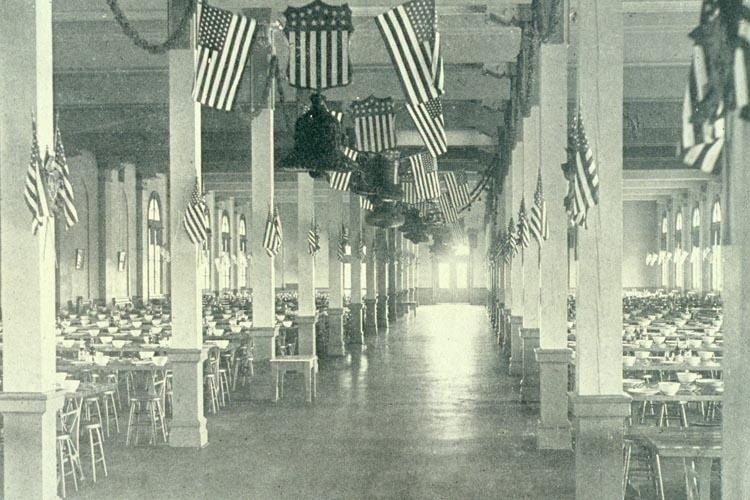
[119,252,127,272]
[76,248,85,270]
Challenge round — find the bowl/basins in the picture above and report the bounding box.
[622,309,722,396]
[203,305,252,349]
[55,309,169,392]
[275,300,297,328]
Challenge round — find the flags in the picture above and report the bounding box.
[192,3,258,113]
[674,2,750,171]
[262,214,280,259]
[563,124,598,226]
[375,1,444,105]
[184,178,210,249]
[332,102,473,264]
[305,227,317,258]
[487,189,546,262]
[53,125,80,231]
[24,116,47,237]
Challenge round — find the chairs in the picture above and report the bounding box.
[46,286,369,498]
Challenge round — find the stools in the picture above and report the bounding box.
[555,289,724,500]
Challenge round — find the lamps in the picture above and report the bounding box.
[283,90,450,262]
